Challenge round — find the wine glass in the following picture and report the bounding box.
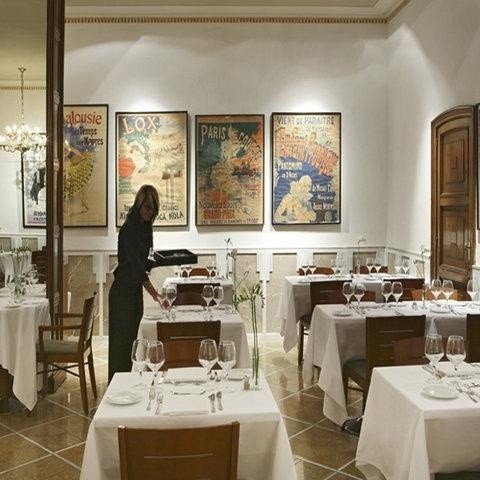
[355,282,365,311]
[217,340,236,394]
[342,282,354,312]
[366,257,374,278]
[131,339,149,390]
[394,259,402,278]
[176,264,193,281]
[147,341,165,390]
[424,334,443,385]
[338,258,345,279]
[202,285,214,314]
[157,285,167,314]
[466,280,478,309]
[402,259,410,278]
[213,286,224,316]
[446,335,465,388]
[308,260,317,280]
[300,258,308,279]
[5,263,39,307]
[380,281,392,311]
[442,280,455,311]
[430,279,442,311]
[166,283,178,315]
[331,259,338,279]
[206,261,220,280]
[392,282,403,311]
[198,339,219,392]
[373,258,382,278]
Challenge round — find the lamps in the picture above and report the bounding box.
[0,68,47,154]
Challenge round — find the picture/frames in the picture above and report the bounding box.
[272,112,341,225]
[195,114,264,226]
[115,110,188,228]
[21,103,109,229]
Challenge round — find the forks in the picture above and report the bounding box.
[154,392,164,415]
[146,387,156,412]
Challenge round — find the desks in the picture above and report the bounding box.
[78,267,479,480]
[0,283,71,411]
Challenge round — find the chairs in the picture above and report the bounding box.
[35,290,99,415]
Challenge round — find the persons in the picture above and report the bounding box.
[107,184,172,385]
[273,175,317,224]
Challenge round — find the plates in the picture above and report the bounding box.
[331,310,351,316]
[145,314,165,321]
[422,385,459,400]
[105,390,143,405]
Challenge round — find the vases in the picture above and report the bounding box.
[10,246,32,304]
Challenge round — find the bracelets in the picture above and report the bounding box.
[147,284,152,292]
